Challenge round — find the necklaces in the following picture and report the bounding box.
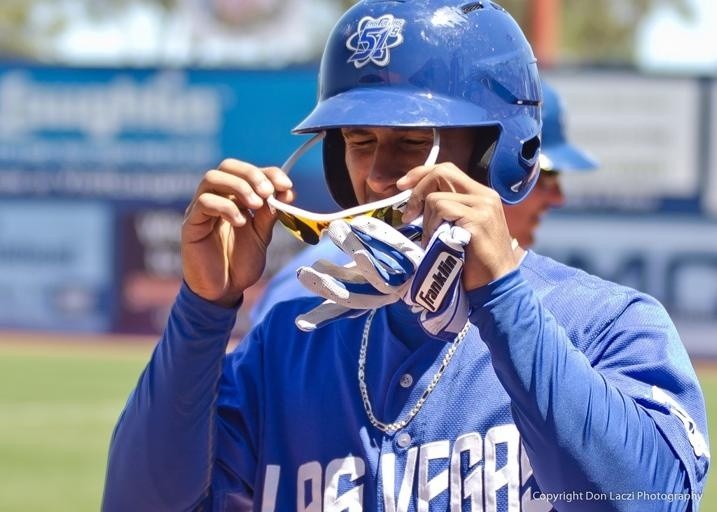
[358,308,473,435]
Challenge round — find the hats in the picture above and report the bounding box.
[540,80,598,172]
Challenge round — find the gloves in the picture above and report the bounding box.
[294,216,470,343]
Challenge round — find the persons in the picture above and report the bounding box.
[241,77,599,330]
[96,2,712,512]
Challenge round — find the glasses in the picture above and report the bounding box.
[265,128,440,244]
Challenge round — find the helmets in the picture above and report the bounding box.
[291,1,542,210]
